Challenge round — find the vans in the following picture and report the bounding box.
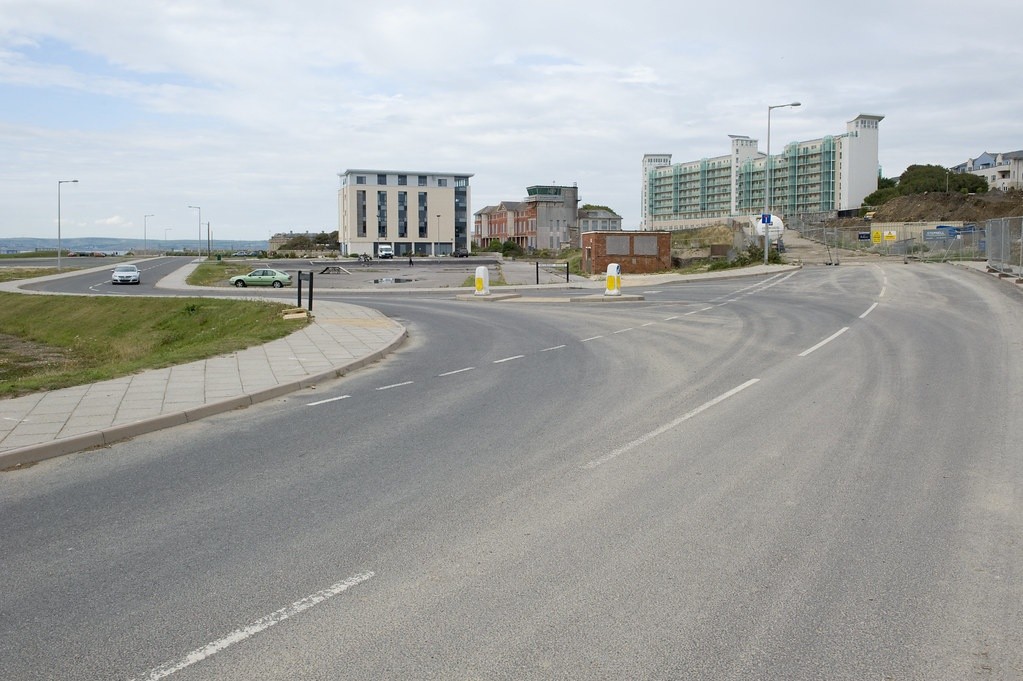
[378,244,394,259]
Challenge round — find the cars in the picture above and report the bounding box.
[67,252,77,257]
[110,265,141,286]
[94,252,106,257]
[453,248,469,257]
[228,268,293,288]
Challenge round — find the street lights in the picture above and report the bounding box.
[436,214,440,257]
[56,179,79,270]
[144,214,155,257]
[164,227,173,255]
[187,205,201,260]
[764,101,804,267]
[201,221,212,260]
